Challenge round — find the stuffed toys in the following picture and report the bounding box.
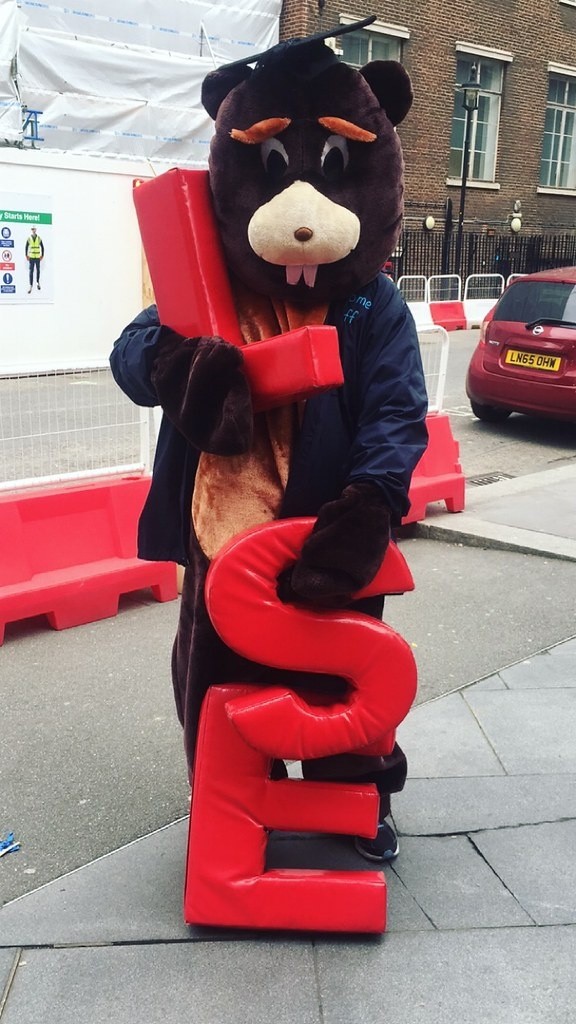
[108,15,430,859]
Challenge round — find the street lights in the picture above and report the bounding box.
[449,59,483,302]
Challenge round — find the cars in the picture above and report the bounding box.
[466,265,576,425]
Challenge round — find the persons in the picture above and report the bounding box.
[25,225,45,294]
